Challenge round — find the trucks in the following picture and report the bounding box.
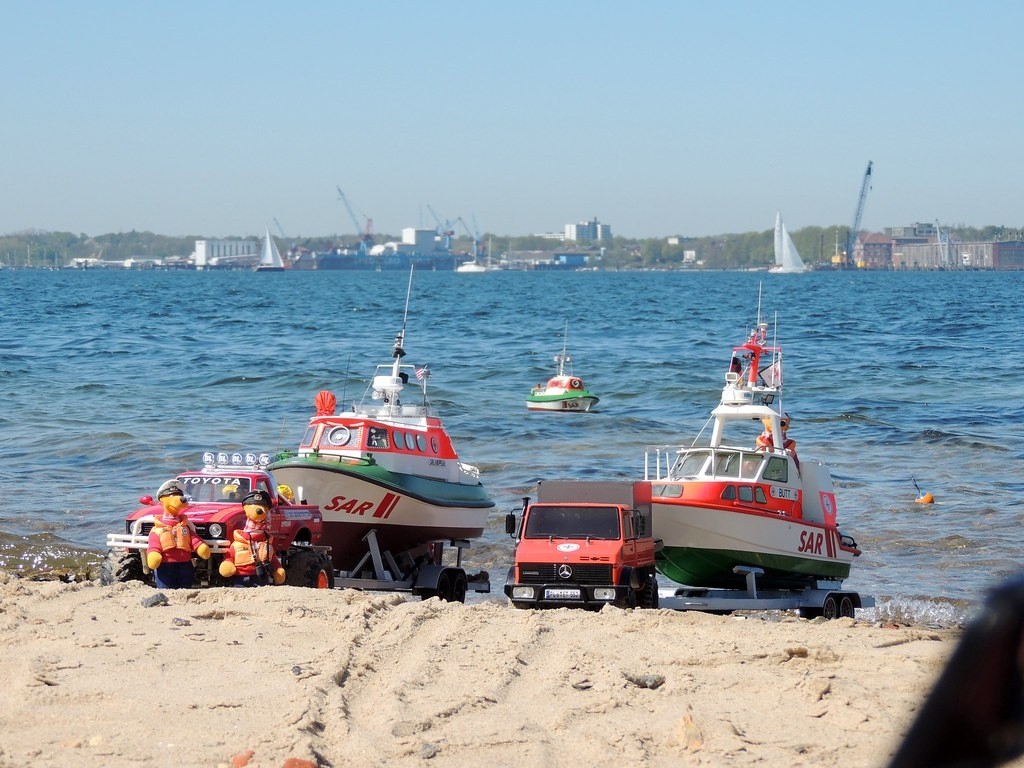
[501,478,665,616]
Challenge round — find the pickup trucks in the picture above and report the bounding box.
[97,451,324,583]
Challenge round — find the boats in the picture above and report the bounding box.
[523,318,604,415]
[644,282,865,590]
[258,261,498,567]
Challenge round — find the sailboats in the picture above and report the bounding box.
[255,225,285,271]
[767,212,814,274]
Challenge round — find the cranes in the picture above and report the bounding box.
[336,185,377,244]
[843,159,875,260]
[426,204,472,242]
